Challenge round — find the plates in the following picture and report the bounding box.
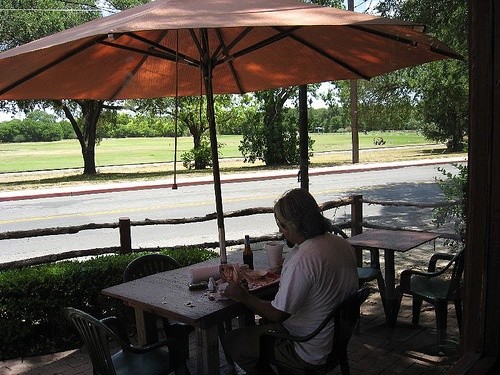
[207,272,280,301]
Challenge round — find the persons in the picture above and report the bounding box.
[222,188,359,375]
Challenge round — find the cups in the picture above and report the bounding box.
[265,242,283,269]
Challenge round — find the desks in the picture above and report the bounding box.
[102,251,281,375]
[343,228,443,342]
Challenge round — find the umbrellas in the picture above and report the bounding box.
[0,0,466,264]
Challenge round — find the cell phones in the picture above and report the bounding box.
[188,281,208,291]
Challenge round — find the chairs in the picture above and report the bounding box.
[386,246,466,347]
[327,225,391,336]
[124,254,233,366]
[246,287,370,375]
[63,306,190,374]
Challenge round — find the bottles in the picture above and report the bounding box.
[208,277,216,292]
[243,235,254,270]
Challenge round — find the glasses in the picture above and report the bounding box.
[276,219,293,230]
